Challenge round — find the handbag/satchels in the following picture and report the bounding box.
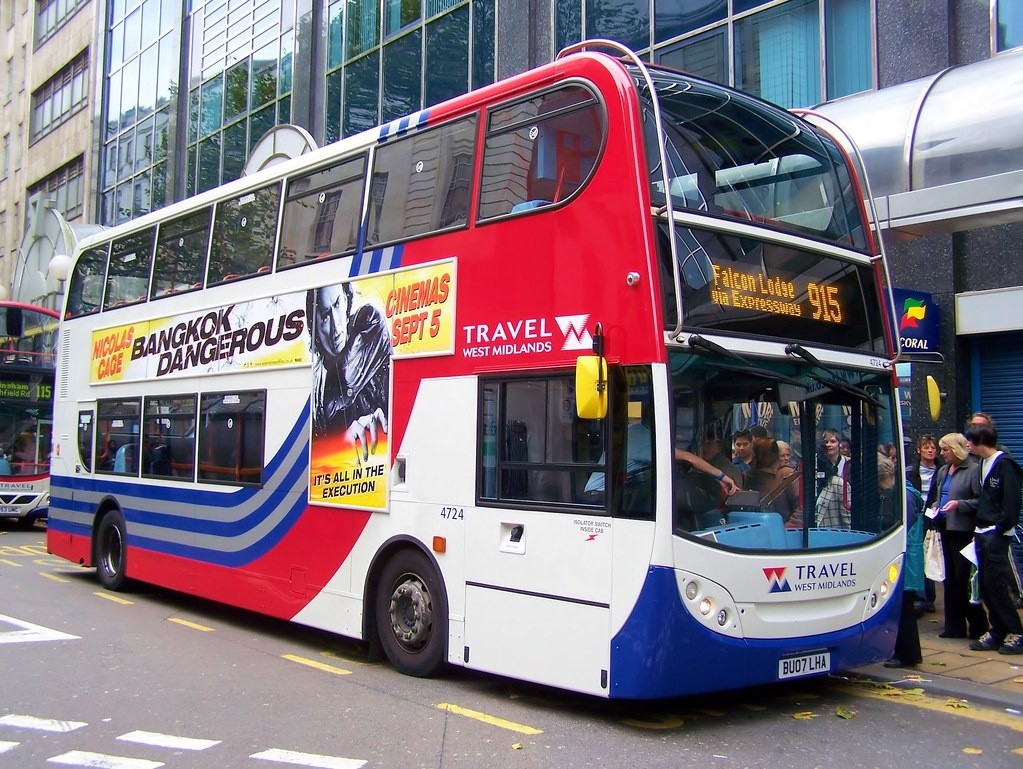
[923,516,946,583]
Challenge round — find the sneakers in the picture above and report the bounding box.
[969,631,1022,654]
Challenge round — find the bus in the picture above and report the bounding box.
[0,302,61,526]
[45,38,948,701]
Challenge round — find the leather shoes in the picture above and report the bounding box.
[885,656,903,667]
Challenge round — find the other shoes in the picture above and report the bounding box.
[912,602,935,619]
[932,627,982,641]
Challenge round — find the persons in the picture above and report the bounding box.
[966,424,1023,655]
[306,282,393,467]
[931,433,990,640]
[13,432,43,476]
[874,458,924,669]
[79,440,118,466]
[580,386,899,541]
[905,434,943,617]
[964,412,1023,608]
